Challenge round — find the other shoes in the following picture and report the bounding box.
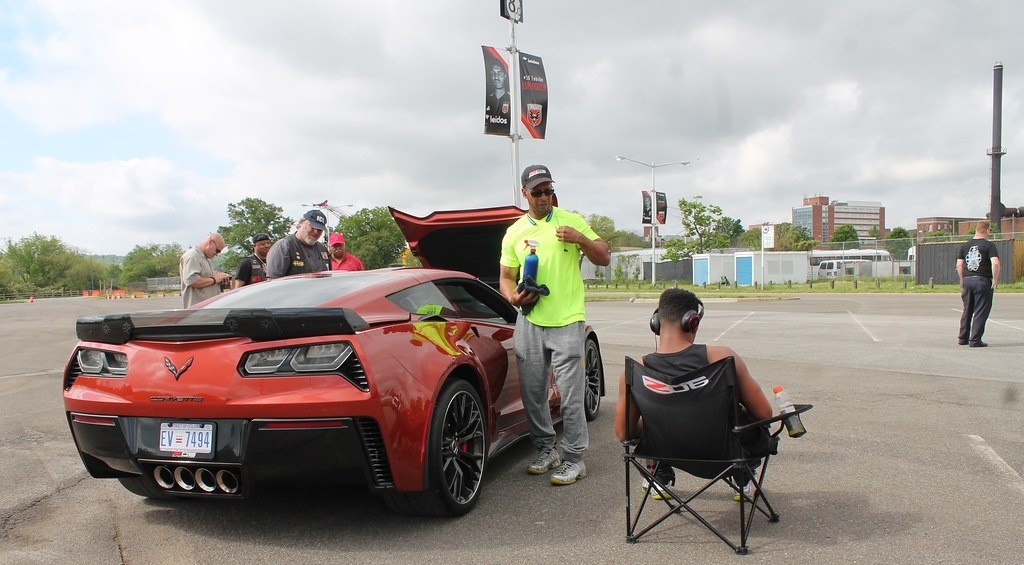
[969,341,988,347]
[958,339,969,345]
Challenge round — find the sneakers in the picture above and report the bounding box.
[733,482,754,502]
[527,448,561,473]
[642,477,673,499]
[551,461,587,484]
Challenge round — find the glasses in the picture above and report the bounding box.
[531,189,554,198]
[215,242,221,253]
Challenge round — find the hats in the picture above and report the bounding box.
[521,164,555,188]
[303,209,327,232]
[252,233,270,244]
[330,233,345,246]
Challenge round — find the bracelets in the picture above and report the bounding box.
[210,276,216,285]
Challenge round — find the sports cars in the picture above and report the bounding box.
[61,204,608,525]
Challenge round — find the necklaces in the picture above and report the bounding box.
[253,253,266,266]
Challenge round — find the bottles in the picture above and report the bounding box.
[773,385,804,435]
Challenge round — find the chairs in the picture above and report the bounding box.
[621,356,779,555]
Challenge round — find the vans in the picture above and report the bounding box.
[816,259,873,281]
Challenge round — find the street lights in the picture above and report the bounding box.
[614,156,691,288]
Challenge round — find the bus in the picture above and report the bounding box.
[808,247,895,284]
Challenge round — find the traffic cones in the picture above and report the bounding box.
[29,297,34,303]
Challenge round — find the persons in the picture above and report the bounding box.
[643,196,652,223]
[179,233,232,309]
[381,304,508,405]
[330,232,365,271]
[500,165,610,484]
[486,57,510,130]
[956,220,1000,347]
[234,233,271,290]
[266,210,330,282]
[614,288,773,501]
